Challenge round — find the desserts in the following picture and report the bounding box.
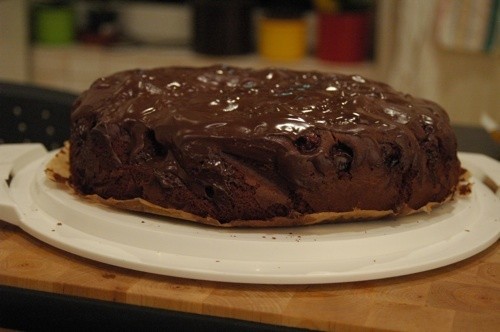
[64,61,471,226]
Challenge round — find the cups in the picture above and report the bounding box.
[251,12,311,64]
[35,9,73,45]
[308,14,368,65]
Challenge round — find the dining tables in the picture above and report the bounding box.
[0,145,500,332]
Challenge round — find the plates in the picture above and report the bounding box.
[0,143,500,285]
